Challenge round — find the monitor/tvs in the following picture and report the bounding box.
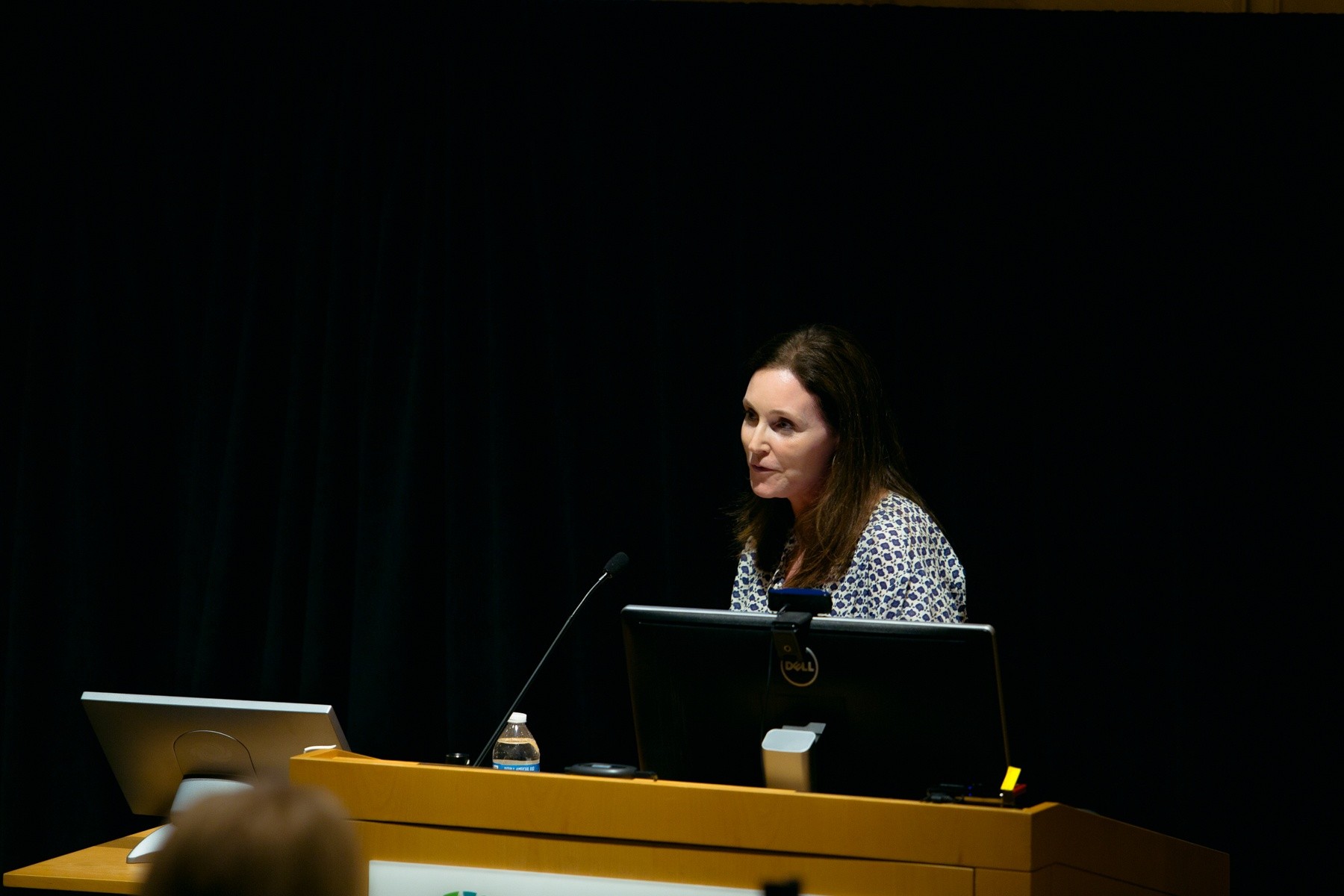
[80,691,350,864]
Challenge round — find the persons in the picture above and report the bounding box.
[133,769,370,896]
[729,322,967,625]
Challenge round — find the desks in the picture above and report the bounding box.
[3,825,187,896]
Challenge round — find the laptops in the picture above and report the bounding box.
[621,604,1010,797]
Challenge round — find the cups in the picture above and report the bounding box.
[445,752,471,769]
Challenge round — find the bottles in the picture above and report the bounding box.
[492,711,540,773]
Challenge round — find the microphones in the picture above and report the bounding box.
[473,551,630,767]
